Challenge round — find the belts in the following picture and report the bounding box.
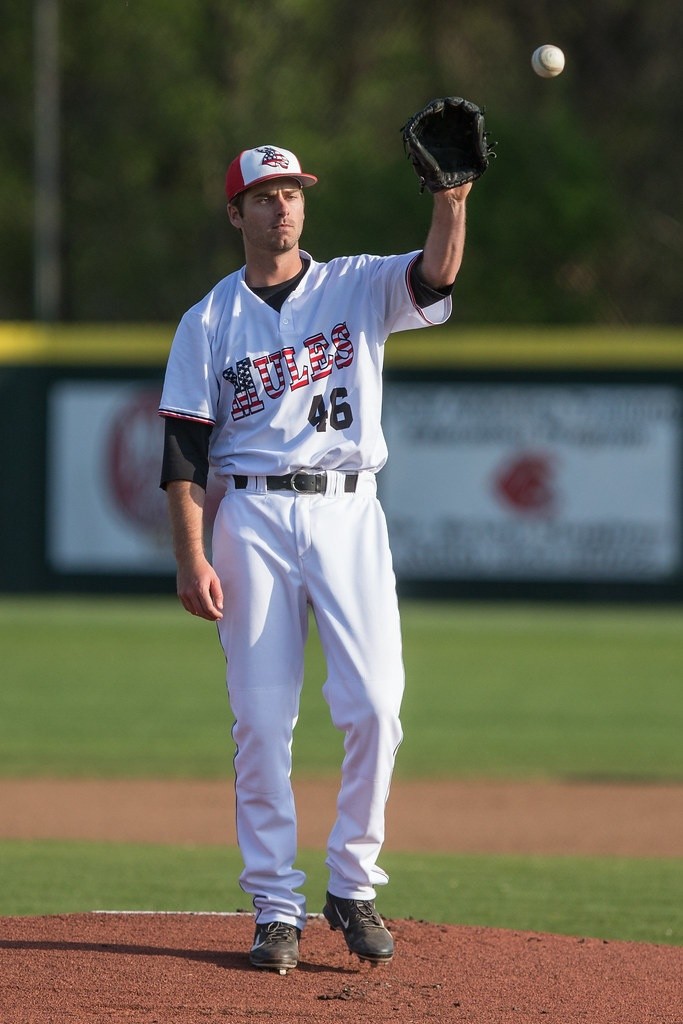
[234,470,356,494]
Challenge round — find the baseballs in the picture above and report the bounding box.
[530,43,565,79]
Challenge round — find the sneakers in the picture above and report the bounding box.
[250,921,301,968]
[323,891,394,966]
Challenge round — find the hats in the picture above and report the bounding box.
[225,145,317,202]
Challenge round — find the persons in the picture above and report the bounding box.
[158,97,491,972]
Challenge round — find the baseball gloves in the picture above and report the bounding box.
[402,96,492,196]
